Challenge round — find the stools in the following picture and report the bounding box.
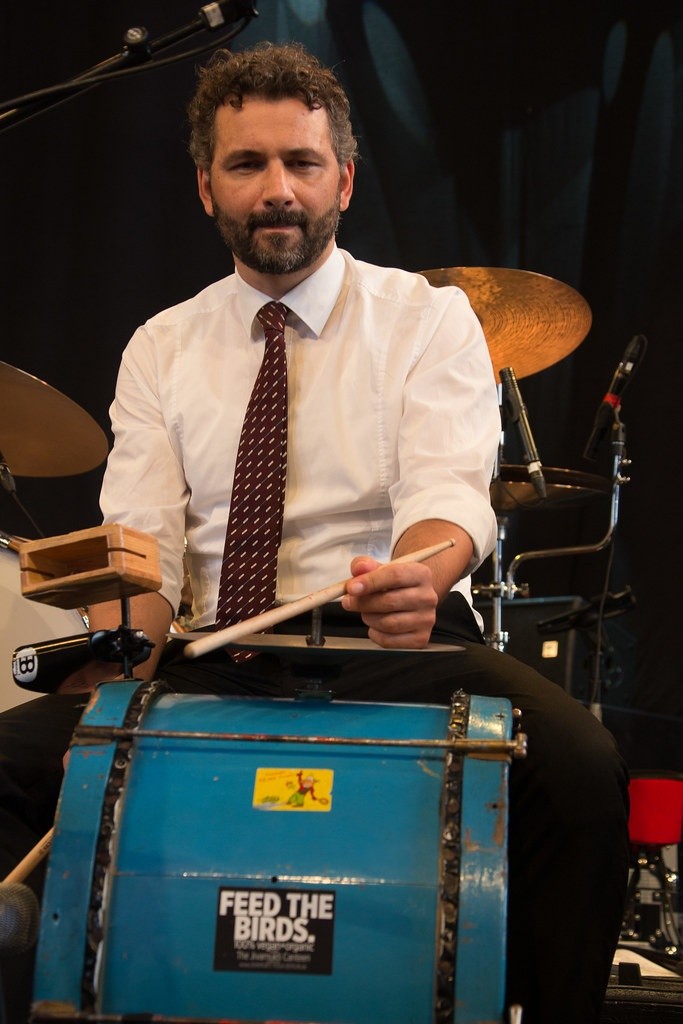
[616,779,683,957]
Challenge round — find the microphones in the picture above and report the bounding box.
[499,366,547,499]
[581,334,647,464]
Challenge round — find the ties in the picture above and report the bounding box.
[215,300,291,663]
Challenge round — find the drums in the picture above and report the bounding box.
[33,676,527,1024]
[0,530,91,713]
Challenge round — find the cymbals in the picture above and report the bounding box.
[0,359,109,478]
[166,631,465,652]
[409,266,592,384]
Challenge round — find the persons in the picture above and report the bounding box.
[0,44,632,1024]
